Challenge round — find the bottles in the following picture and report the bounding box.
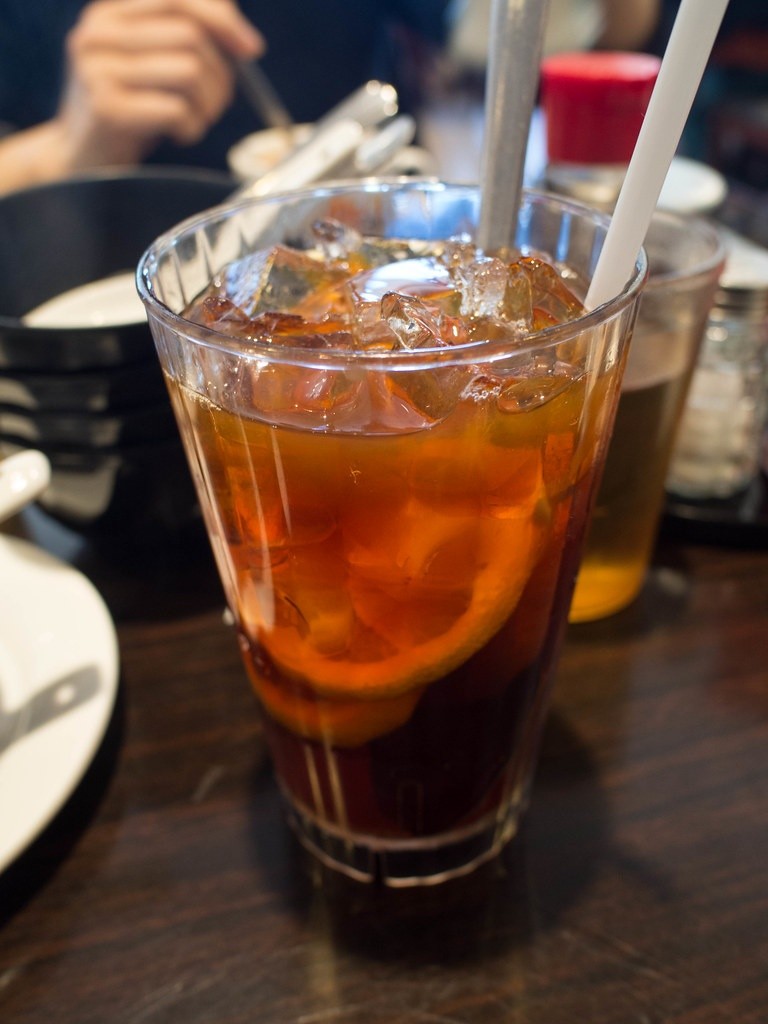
[522,53,661,237]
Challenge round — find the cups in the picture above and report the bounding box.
[566,204,729,624]
[134,174,649,890]
[228,124,436,192]
[1,172,244,626]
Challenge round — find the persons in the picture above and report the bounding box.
[1,1,425,201]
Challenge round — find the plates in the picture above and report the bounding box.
[0,533,122,875]
